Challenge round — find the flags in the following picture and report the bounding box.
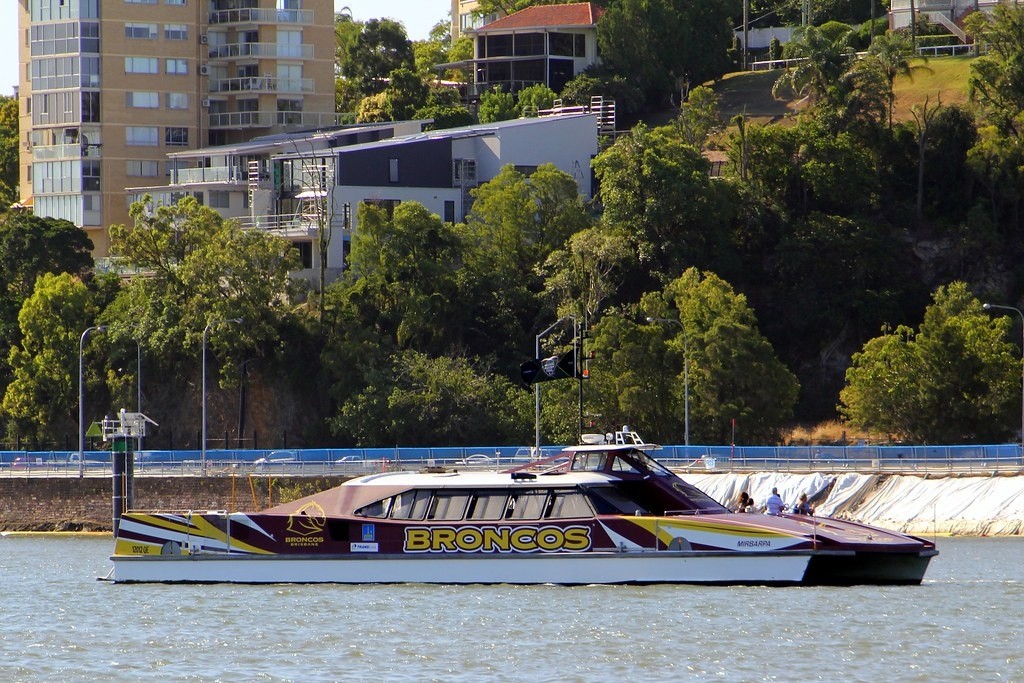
[520,348,578,384]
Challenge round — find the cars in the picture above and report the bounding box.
[335,455,360,466]
[12,458,53,468]
[455,454,497,465]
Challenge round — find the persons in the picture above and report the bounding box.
[745,498,758,513]
[737,492,749,513]
[765,487,785,517]
[792,493,813,516]
[592,454,606,470]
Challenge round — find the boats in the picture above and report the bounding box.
[95,422,942,589]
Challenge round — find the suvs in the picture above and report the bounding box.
[254,450,298,466]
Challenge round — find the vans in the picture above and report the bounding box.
[515,448,564,464]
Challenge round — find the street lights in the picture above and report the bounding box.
[534,312,577,466]
[646,316,690,468]
[95,326,143,453]
[77,327,106,478]
[979,303,1023,341]
[201,318,243,475]
